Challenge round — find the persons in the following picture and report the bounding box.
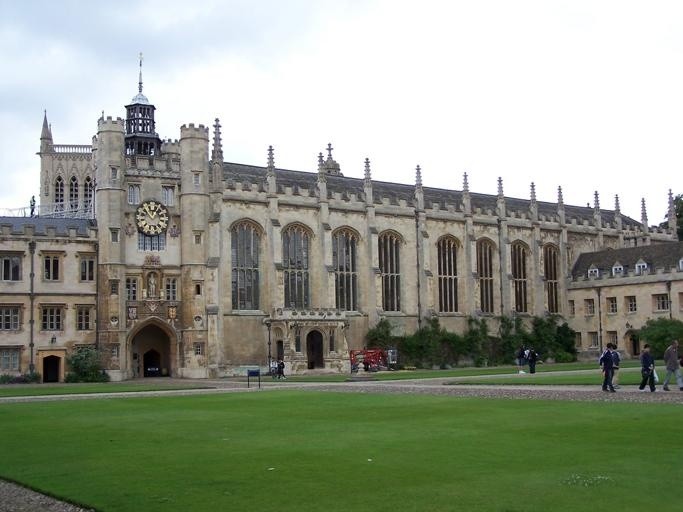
[610,344,621,390]
[276,358,286,380]
[662,340,683,390]
[637,343,659,393]
[598,342,616,392]
[269,356,278,379]
[517,344,527,375]
[526,347,538,374]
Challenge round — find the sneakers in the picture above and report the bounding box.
[603,385,683,392]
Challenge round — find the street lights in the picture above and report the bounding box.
[264,319,271,375]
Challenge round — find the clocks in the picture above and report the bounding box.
[134,200,169,237]
